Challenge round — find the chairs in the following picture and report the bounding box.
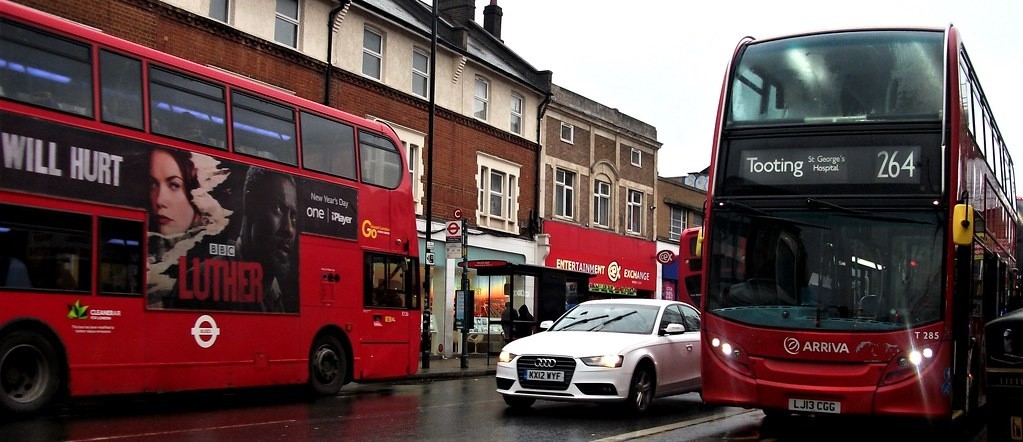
[468,333,487,357]
[860,295,878,318]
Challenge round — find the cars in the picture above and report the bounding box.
[983,310,1023,419]
[495,299,701,419]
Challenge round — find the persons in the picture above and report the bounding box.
[162,166,298,313]
[0,229,35,288]
[517,304,535,339]
[147,145,229,308]
[501,302,518,344]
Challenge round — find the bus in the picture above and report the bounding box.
[680,228,703,311]
[0,0,422,421]
[700,24,1016,437]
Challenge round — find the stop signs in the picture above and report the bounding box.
[446,221,462,236]
